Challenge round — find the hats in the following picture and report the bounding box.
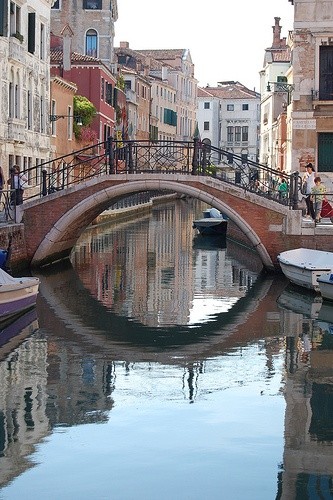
[305,162,313,168]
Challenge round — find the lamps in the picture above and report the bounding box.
[267,82,294,91]
[50,113,83,126]
[275,138,291,148]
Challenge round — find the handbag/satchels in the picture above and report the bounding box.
[301,181,307,194]
[21,183,29,190]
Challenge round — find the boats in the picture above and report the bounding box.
[0,305,40,364]
[0,235,42,318]
[277,247,333,300]
[191,207,229,236]
[275,282,333,324]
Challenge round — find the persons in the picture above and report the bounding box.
[311,177,328,223]
[277,179,287,197]
[302,163,319,220]
[8,165,28,205]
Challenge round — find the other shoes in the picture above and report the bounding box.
[316,219,319,224]
